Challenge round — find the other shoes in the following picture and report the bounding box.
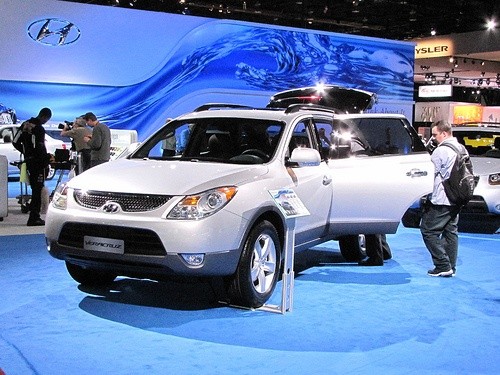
[27,218,45,226]
[359,261,381,266]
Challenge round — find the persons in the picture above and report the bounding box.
[61,112,111,174]
[421,119,473,278]
[12,108,52,226]
[162,117,194,160]
[358,232,393,266]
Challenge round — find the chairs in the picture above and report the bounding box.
[200,133,238,161]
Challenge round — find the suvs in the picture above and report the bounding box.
[45,85,435,309]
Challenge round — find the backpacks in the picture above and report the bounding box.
[436,143,479,205]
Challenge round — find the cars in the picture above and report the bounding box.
[404,121,500,227]
[0,124,73,180]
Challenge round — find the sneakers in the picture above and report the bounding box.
[428,269,453,276]
[452,267,456,276]
